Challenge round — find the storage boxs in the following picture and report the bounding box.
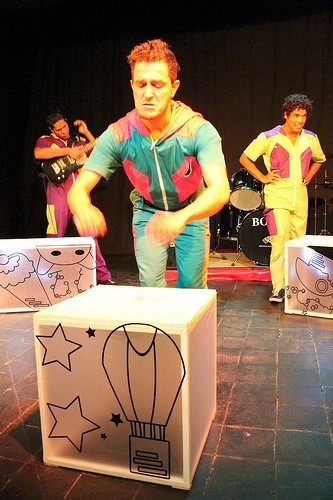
[284,235,333,319]
[0,236,97,313]
[33,285,218,491]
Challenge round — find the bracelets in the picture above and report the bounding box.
[302,178,307,184]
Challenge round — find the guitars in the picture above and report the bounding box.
[42,136,100,186]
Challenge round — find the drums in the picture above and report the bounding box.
[216,203,256,240]
[235,208,272,266]
[228,168,263,212]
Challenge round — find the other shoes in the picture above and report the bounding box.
[103,278,119,285]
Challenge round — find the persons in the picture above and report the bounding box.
[239,94,326,303]
[66,39,230,289]
[34,113,117,286]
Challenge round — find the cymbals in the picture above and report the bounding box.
[308,180,333,187]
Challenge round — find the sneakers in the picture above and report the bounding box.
[269,288,285,302]
